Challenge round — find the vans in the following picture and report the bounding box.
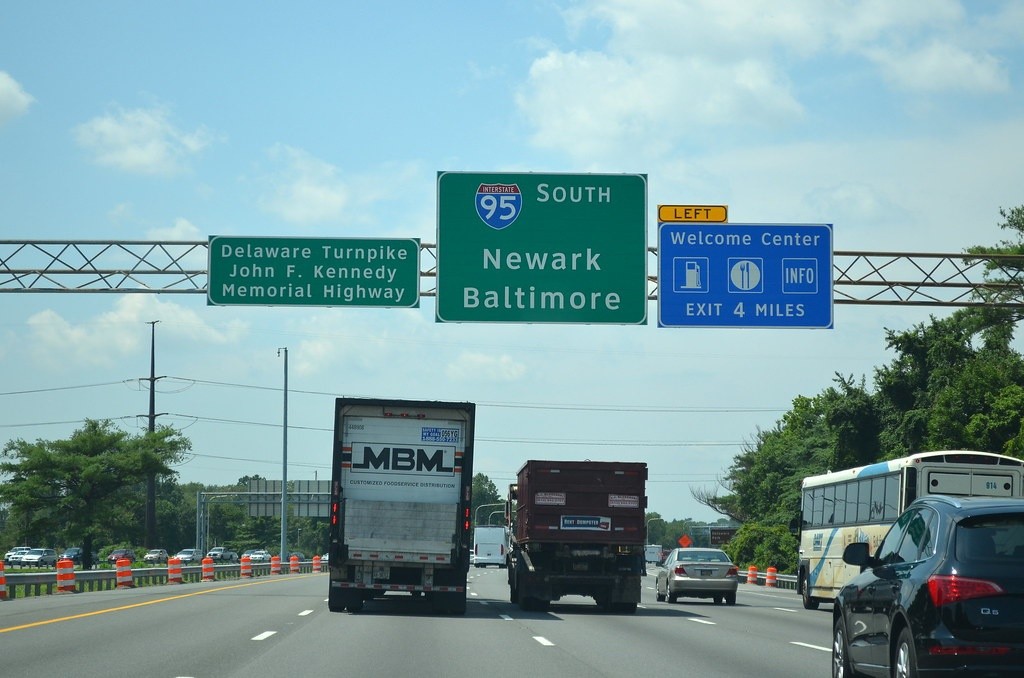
[21,549,58,567]
[176,549,203,563]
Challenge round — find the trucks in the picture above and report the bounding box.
[473,527,508,568]
[503,460,648,611]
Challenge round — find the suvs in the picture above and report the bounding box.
[8,551,29,566]
[831,494,1024,678]
[4,547,31,562]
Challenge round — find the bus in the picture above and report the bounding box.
[790,448,1024,610]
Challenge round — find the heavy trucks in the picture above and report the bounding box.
[329,397,476,616]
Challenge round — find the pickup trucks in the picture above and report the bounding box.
[58,547,100,565]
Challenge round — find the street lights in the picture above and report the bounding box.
[682,520,694,536]
[298,528,302,548]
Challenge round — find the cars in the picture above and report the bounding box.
[469,550,474,564]
[655,548,739,605]
[107,549,136,563]
[143,548,169,563]
[321,553,328,560]
[206,547,239,561]
[277,552,305,562]
[241,549,272,562]
